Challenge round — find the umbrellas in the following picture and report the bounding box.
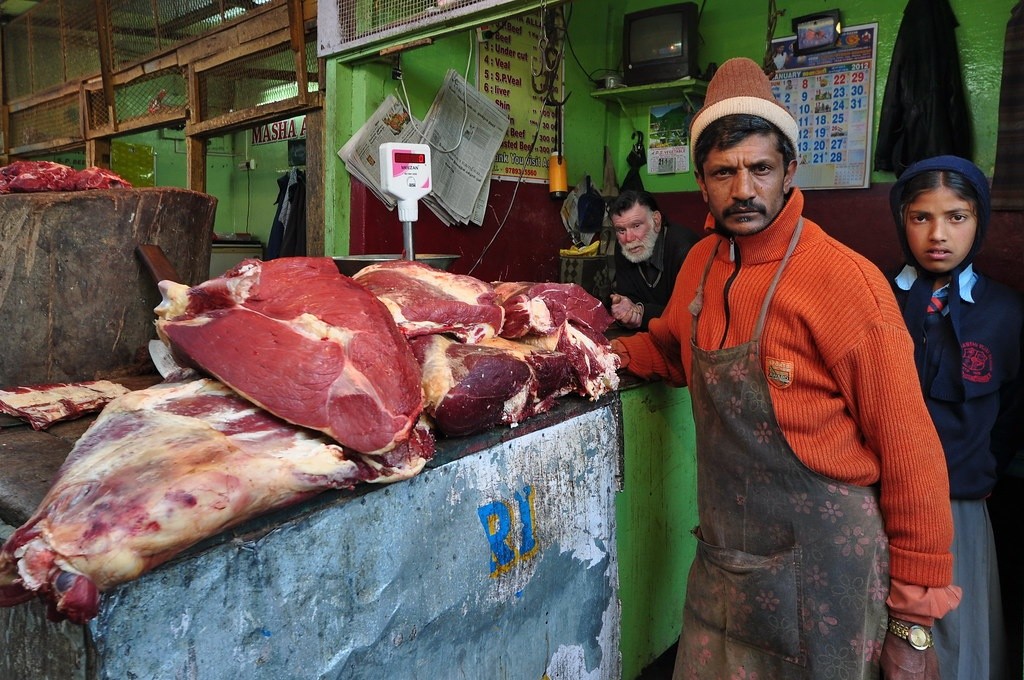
[618,130,647,194]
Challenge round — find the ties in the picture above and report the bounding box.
[924,296,948,330]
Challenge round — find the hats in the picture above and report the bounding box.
[690,57,799,165]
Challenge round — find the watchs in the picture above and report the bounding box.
[887,619,936,651]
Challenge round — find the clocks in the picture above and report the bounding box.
[791,8,841,57]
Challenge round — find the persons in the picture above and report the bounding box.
[604,190,701,333]
[884,155,1024,680]
[799,28,829,50]
[772,43,794,70]
[608,57,963,680]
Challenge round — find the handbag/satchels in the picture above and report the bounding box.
[577,175,604,233]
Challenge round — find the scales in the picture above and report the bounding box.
[330,141,463,277]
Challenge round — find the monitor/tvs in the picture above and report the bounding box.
[623,2,699,86]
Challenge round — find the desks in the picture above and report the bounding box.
[0,316,699,680]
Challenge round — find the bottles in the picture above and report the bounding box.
[548,151,568,201]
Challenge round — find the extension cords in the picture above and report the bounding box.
[476,25,491,42]
